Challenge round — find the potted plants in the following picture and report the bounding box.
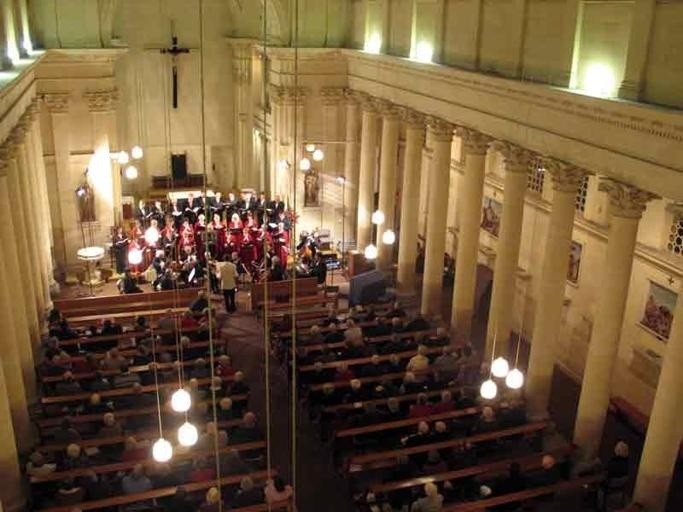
[635,279,679,345]
[566,238,585,287]
[479,193,503,240]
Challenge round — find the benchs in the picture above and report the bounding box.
[30,288,303,512]
[255,287,628,512]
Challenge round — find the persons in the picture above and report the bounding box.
[269,305,629,512]
[26,392,292,510]
[79,181,96,222]
[112,189,327,294]
[306,168,318,202]
[215,253,239,313]
[34,290,238,392]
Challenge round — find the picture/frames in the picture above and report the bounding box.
[635,279,679,345]
[566,238,585,287]
[76,246,104,285]
[479,193,503,240]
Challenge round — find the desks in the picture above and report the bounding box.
[76,246,104,285]
